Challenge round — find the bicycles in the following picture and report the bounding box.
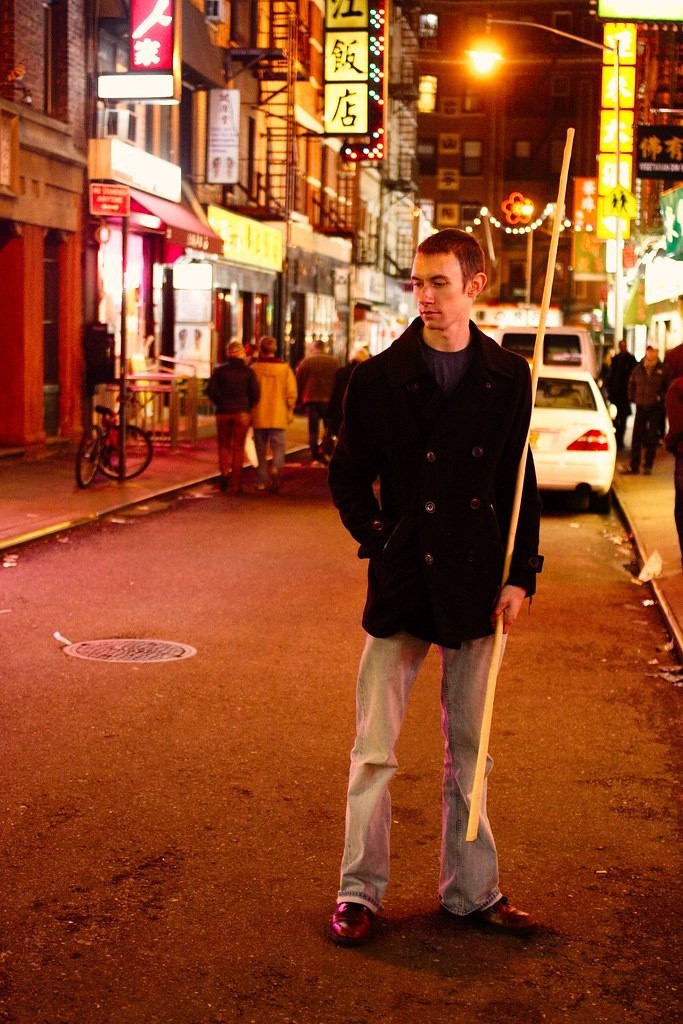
[75,387,153,489]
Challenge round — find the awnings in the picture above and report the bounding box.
[623,277,654,327]
[87,183,224,255]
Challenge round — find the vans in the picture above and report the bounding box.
[499,328,598,383]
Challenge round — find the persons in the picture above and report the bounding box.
[331,231,545,944]
[205,337,297,492]
[600,340,683,562]
[295,340,371,464]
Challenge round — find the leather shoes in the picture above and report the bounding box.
[439,897,539,932]
[328,902,374,945]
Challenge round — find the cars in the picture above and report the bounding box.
[530,366,619,515]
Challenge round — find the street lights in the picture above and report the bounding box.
[469,13,621,348]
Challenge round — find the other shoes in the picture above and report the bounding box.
[644,468,652,475]
[621,469,638,475]
